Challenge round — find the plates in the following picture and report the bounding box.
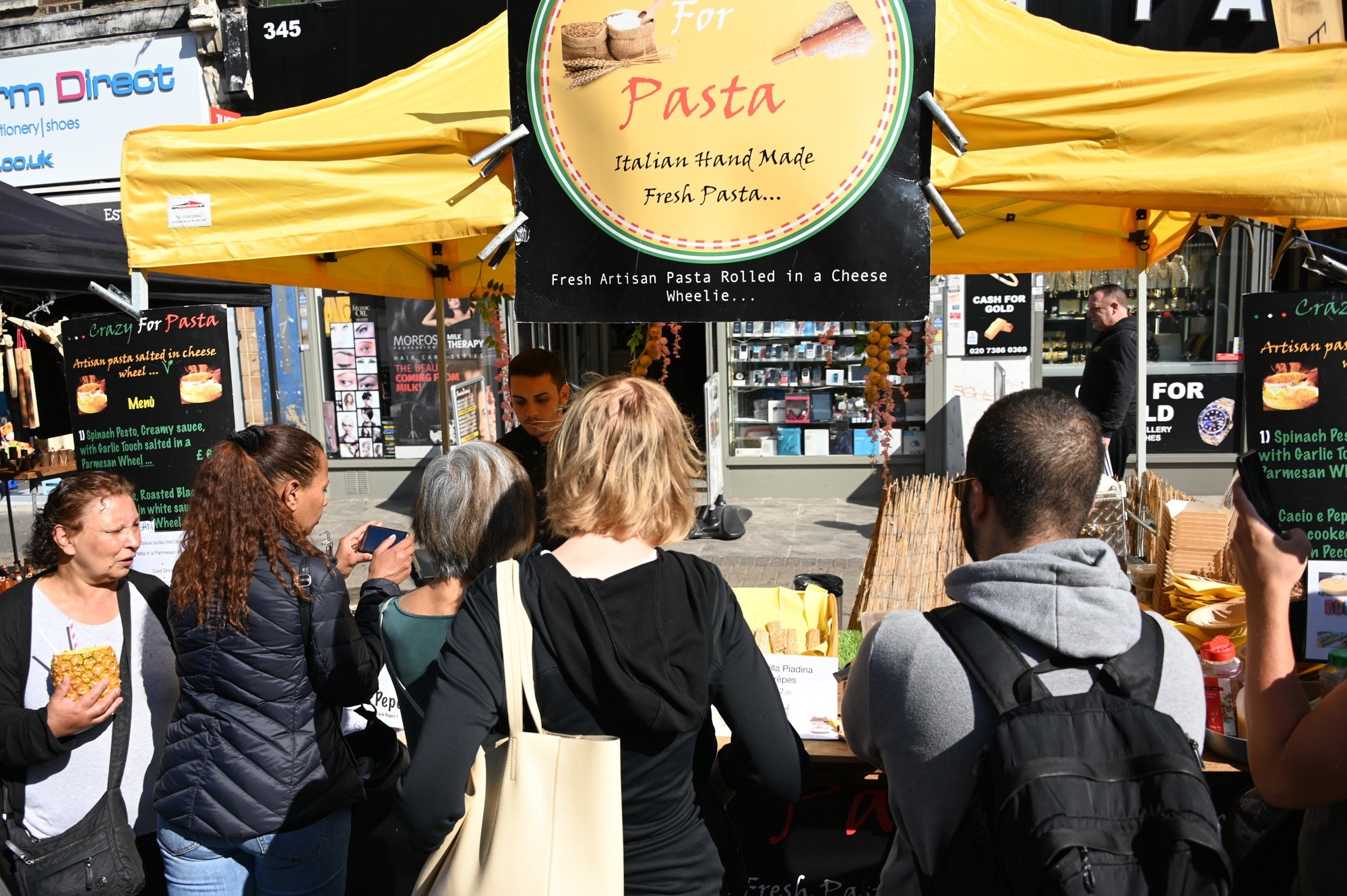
[77,393,107,413]
[1263,386,1319,410]
[1319,576,1347,596]
[180,384,222,404]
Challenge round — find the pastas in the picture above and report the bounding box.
[1264,374,1307,383]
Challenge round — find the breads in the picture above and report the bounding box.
[750,620,825,655]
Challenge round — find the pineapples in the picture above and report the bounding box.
[51,646,121,703]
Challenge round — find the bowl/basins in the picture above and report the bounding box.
[1264,373,1307,389]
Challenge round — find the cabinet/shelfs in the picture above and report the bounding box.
[727,321,926,465]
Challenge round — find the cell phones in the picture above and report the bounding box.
[357,525,410,555]
[1236,449,1283,538]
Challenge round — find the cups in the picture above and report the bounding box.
[1128,563,1155,604]
[77,383,100,396]
[179,372,213,388]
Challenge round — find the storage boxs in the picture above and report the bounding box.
[762,439,777,456]
[749,371,753,385]
[710,586,842,741]
[8,479,18,490]
[1204,730,1250,765]
[804,429,829,455]
[811,394,831,420]
[767,400,786,423]
[825,369,845,386]
[878,427,903,456]
[41,477,62,496]
[853,428,879,455]
[767,382,798,387]
[1152,499,1230,616]
[753,397,774,410]
[776,426,801,456]
[731,322,841,360]
[901,375,914,384]
[828,427,853,455]
[903,427,927,455]
[733,380,746,386]
[753,370,765,386]
[905,399,925,421]
[847,364,874,384]
[914,374,925,384]
[735,447,762,457]
[784,392,811,424]
[753,409,768,420]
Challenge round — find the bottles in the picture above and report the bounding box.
[1200,635,1248,740]
[0,558,38,593]
[1318,649,1347,699]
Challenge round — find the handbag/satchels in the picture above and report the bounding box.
[325,662,411,785]
[6,789,146,895]
[413,557,623,896]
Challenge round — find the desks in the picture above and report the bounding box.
[714,676,869,769]
[0,459,77,533]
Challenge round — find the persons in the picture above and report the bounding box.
[157,425,416,896]
[377,445,538,762]
[1224,479,1346,896]
[489,350,575,550]
[399,374,812,896]
[841,387,1206,896]
[422,298,477,361]
[1,470,167,896]
[1081,284,1139,481]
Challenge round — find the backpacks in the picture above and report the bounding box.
[920,602,1235,896]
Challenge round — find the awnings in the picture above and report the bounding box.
[0,180,283,426]
[120,0,1347,455]
